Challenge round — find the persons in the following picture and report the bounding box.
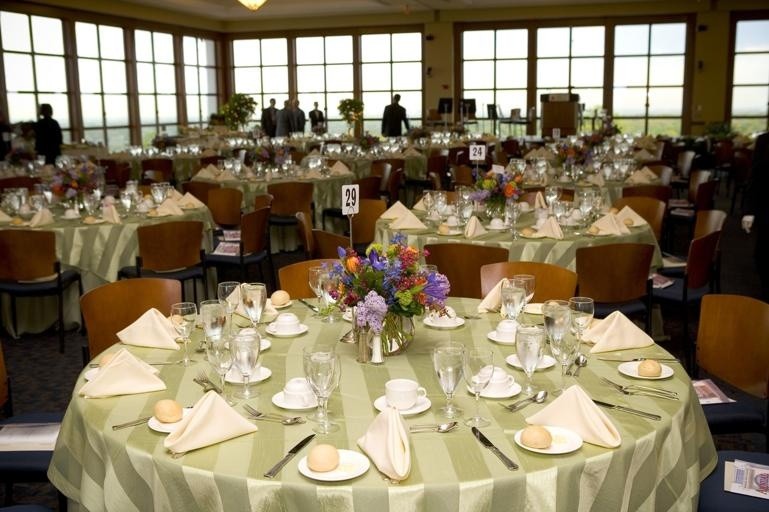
[741,101,769,306]
[381,94,410,137]
[261,98,280,138]
[309,101,324,132]
[34,103,63,167]
[275,100,292,137]
[288,100,306,133]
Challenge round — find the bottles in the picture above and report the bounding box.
[356,327,371,363]
[370,329,384,364]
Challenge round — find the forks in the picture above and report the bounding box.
[242,404,306,424]
[197,368,223,393]
[602,377,679,402]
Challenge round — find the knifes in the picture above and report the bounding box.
[298,297,319,314]
[263,434,318,478]
[590,398,662,422]
[88,363,170,368]
[597,356,676,363]
[471,427,520,471]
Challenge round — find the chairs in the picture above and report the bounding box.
[1,229,83,355]
[479,260,578,305]
[684,293,769,435]
[116,219,209,302]
[204,208,277,284]
[313,229,350,259]
[0,90,769,248]
[278,258,340,299]
[698,449,769,511]
[650,230,722,336]
[80,278,182,362]
[572,242,654,334]
[424,244,510,300]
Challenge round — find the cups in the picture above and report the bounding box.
[495,320,521,341]
[501,279,526,320]
[429,306,457,324]
[482,366,515,394]
[384,379,427,409]
[269,312,301,334]
[283,378,312,407]
[241,283,267,328]
[128,144,207,158]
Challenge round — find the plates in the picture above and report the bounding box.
[224,338,271,352]
[9,222,29,227]
[468,383,523,400]
[265,322,308,338]
[523,302,544,314]
[505,353,557,372]
[513,428,583,454]
[488,330,516,346]
[271,390,329,412]
[266,297,292,308]
[618,361,674,380]
[424,316,465,329]
[222,364,274,384]
[298,449,371,483]
[374,395,432,417]
[84,368,159,380]
[147,408,190,433]
[82,219,105,224]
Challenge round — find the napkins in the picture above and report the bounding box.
[525,385,623,449]
[165,390,258,455]
[580,310,654,354]
[476,278,512,314]
[115,306,181,352]
[355,406,414,481]
[77,349,166,399]
[227,283,279,323]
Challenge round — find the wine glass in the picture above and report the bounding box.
[309,266,329,319]
[218,281,241,341]
[422,184,606,243]
[216,154,328,180]
[228,335,261,398]
[543,300,570,363]
[309,352,343,435]
[433,340,466,418]
[508,157,634,184]
[302,343,337,422]
[203,299,232,362]
[323,143,399,160]
[1,181,175,218]
[200,301,224,360]
[514,324,547,395]
[461,349,495,426]
[548,310,583,396]
[513,272,536,326]
[171,301,198,366]
[207,334,236,407]
[568,296,594,358]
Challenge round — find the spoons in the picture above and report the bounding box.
[410,421,458,432]
[247,417,301,425]
[508,390,548,408]
[193,378,217,393]
[573,355,589,376]
